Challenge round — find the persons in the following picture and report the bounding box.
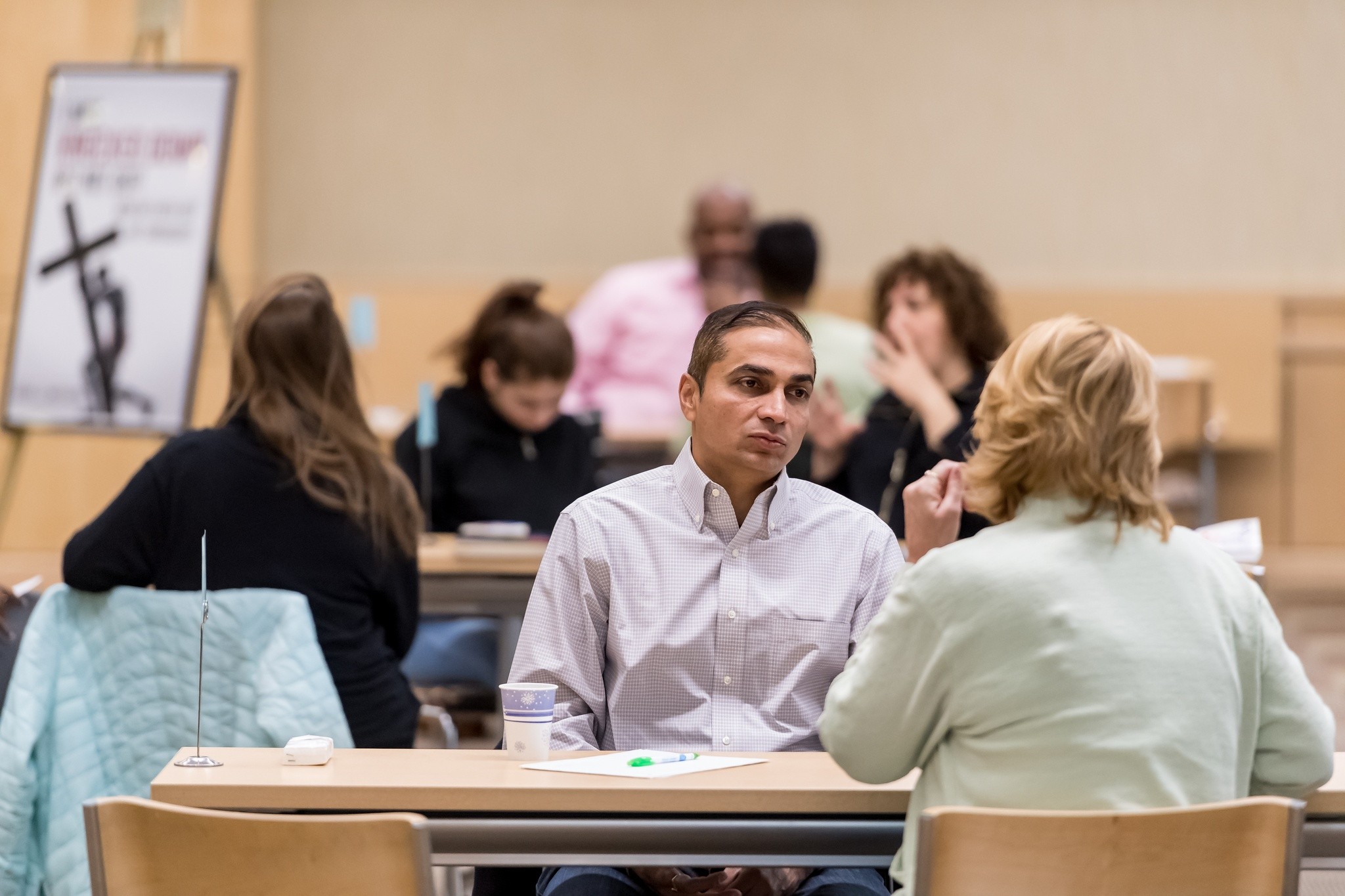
[501,301,905,896]
[817,318,1335,896]
[62,271,423,749]
[379,182,1022,709]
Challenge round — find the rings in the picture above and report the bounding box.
[925,470,945,488]
[672,873,681,893]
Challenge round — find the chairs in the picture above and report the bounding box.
[47,585,307,896]
[913,794,1309,896]
[84,796,437,896]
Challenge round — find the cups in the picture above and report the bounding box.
[498,682,559,762]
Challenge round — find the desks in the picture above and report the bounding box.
[416,533,548,712]
[150,745,1345,896]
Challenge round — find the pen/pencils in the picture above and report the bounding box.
[629,753,699,767]
